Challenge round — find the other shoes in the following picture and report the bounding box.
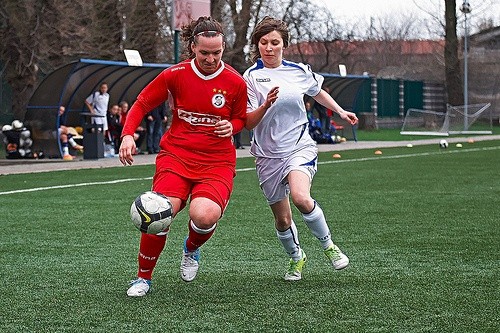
[73,144,84,151]
[63,155,72,161]
[155,150,159,153]
[148,150,154,154]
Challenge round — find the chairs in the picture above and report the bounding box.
[330,120,344,143]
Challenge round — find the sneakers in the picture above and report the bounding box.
[180,235,201,281]
[323,244,349,271]
[285,249,307,281]
[127,278,151,297]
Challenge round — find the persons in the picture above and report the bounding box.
[84,82,110,152]
[119,17,247,297]
[233,132,245,150]
[51,106,84,160]
[305,88,334,139]
[145,104,167,154]
[243,16,358,281]
[107,101,145,155]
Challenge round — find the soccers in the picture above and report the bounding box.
[129,190,174,236]
[1,120,32,157]
[438,138,448,150]
